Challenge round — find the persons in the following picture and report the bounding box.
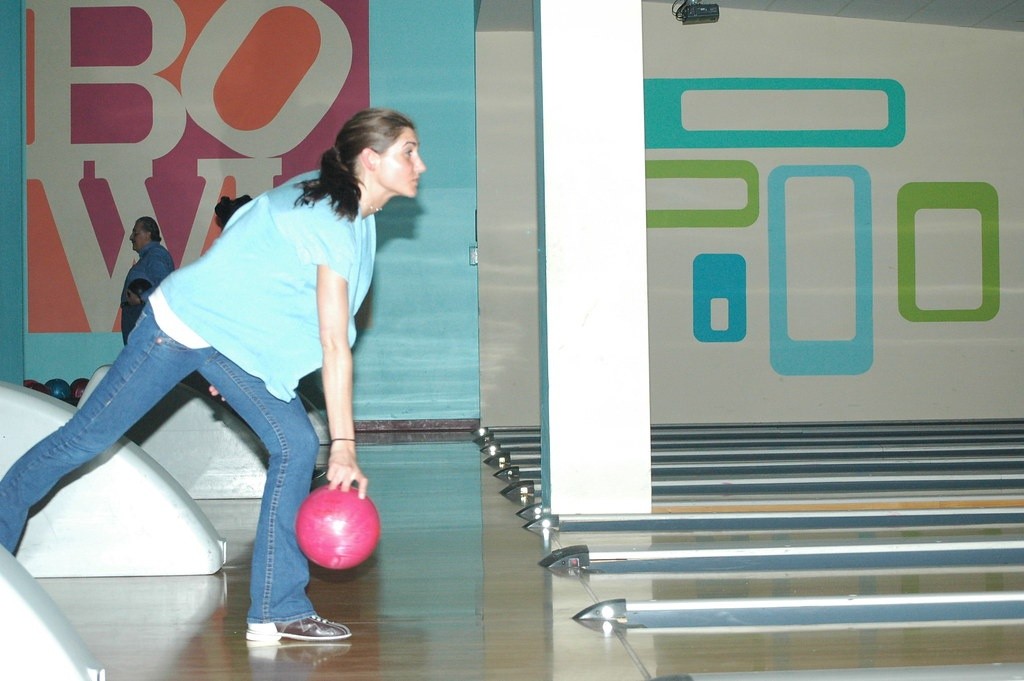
[120,216,173,346]
[0,107,428,643]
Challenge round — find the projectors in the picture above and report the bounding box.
[682,3,720,26]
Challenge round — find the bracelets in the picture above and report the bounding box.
[331,438,356,446]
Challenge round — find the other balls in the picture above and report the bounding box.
[294,484,382,571]
[24,378,90,407]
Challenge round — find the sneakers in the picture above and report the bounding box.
[246,614,353,642]
